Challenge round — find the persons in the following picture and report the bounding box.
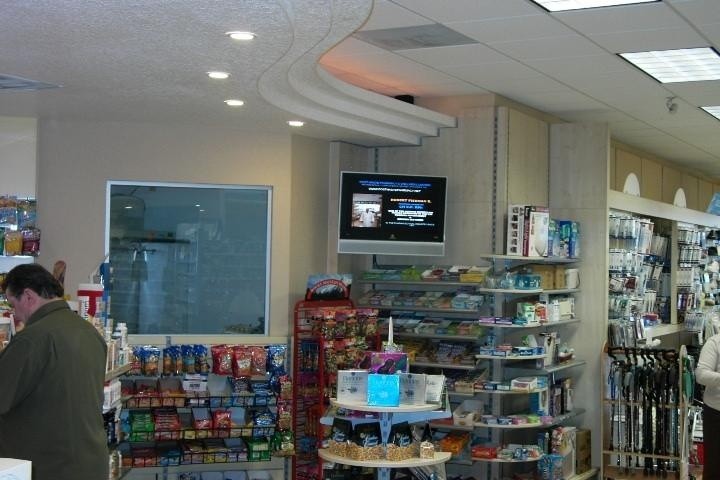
[695,332,720,480]
[359,209,374,227]
[0,263,109,480]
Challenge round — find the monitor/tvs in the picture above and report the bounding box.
[337,172,451,257]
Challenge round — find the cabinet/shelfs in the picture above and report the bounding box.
[121,253,589,480]
[2,193,134,478]
[597,191,719,478]
